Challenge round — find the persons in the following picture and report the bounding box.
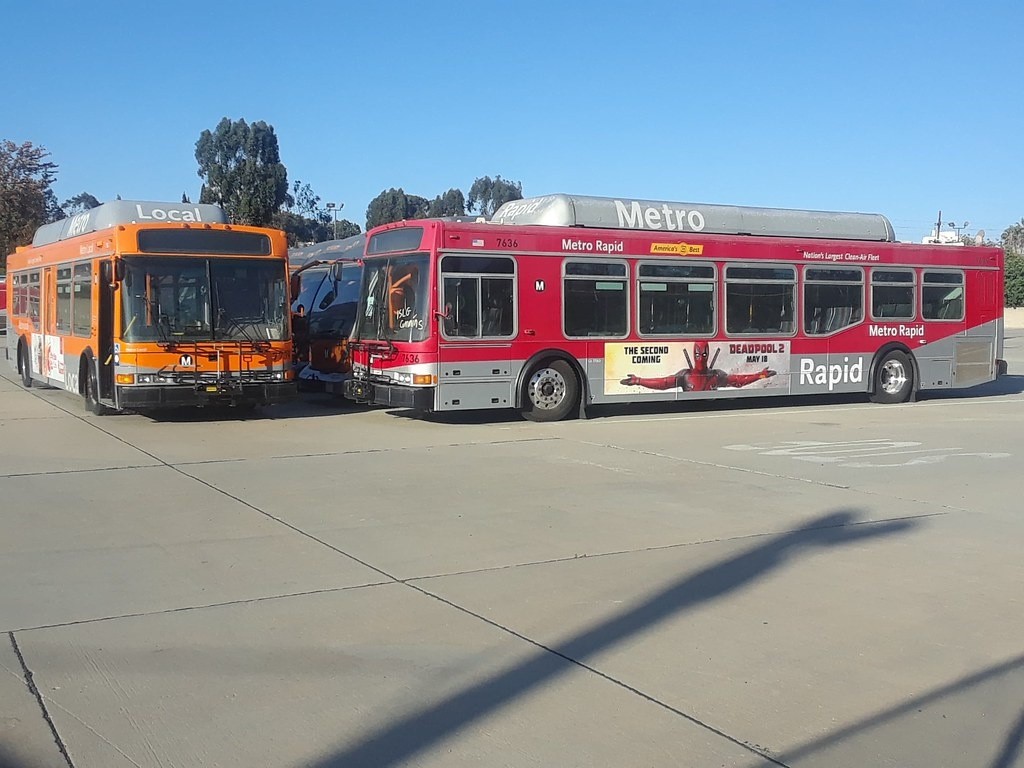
[620,342,777,392]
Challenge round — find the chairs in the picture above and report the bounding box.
[935,304,949,319]
[880,304,895,317]
[946,300,963,319]
[765,328,778,332]
[820,308,836,334]
[810,312,821,333]
[923,303,932,319]
[830,307,852,332]
[742,328,760,333]
[894,304,911,317]
[779,321,793,333]
[482,306,503,335]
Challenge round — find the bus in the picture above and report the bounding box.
[345,193,1007,423]
[287,234,369,392]
[6,201,305,417]
